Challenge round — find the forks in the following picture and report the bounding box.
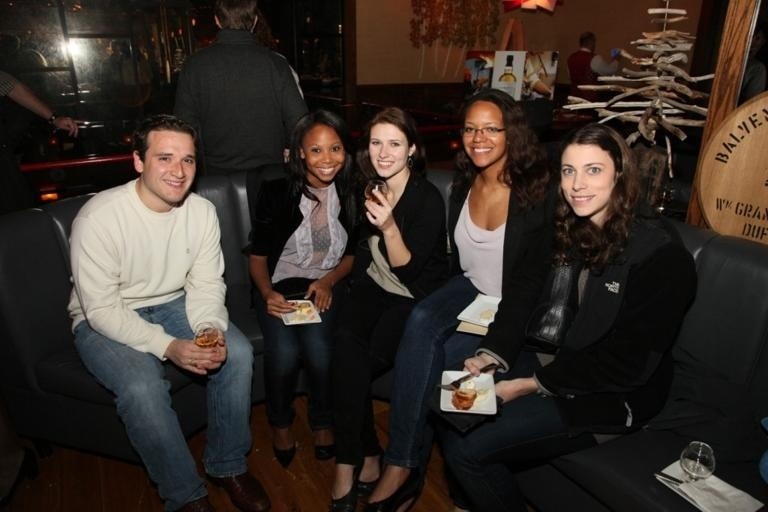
[438,361,498,392]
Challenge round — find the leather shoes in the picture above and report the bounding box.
[187,500,217,512]
[223,475,269,512]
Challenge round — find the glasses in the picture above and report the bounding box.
[458,126,509,138]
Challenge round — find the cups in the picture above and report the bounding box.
[193,321,219,349]
[364,178,388,204]
[679,441,717,484]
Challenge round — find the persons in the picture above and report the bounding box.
[172,0,311,177]
[285,107,454,512]
[244,109,360,467]
[569,33,621,96]
[440,124,700,512]
[67,115,272,512]
[364,89,560,512]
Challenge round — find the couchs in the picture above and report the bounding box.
[0,170,768,512]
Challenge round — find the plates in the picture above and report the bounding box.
[456,294,502,328]
[281,299,322,326]
[440,368,497,416]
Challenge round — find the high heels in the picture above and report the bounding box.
[272,431,296,467]
[357,451,382,497]
[328,463,355,512]
[314,429,334,459]
[364,469,420,512]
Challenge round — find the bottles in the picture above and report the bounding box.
[498,54,517,101]
